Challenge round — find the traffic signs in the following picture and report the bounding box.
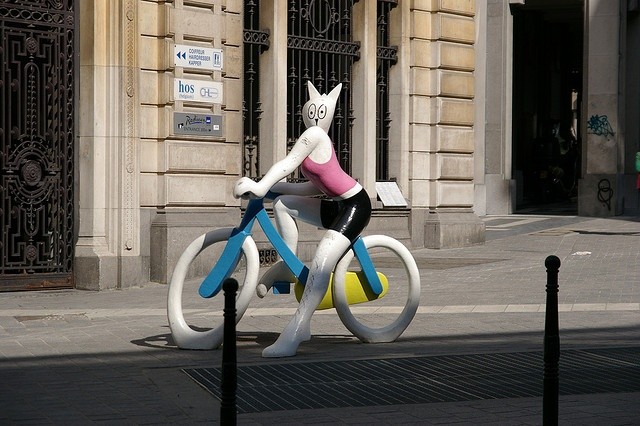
[174,44,223,71]
